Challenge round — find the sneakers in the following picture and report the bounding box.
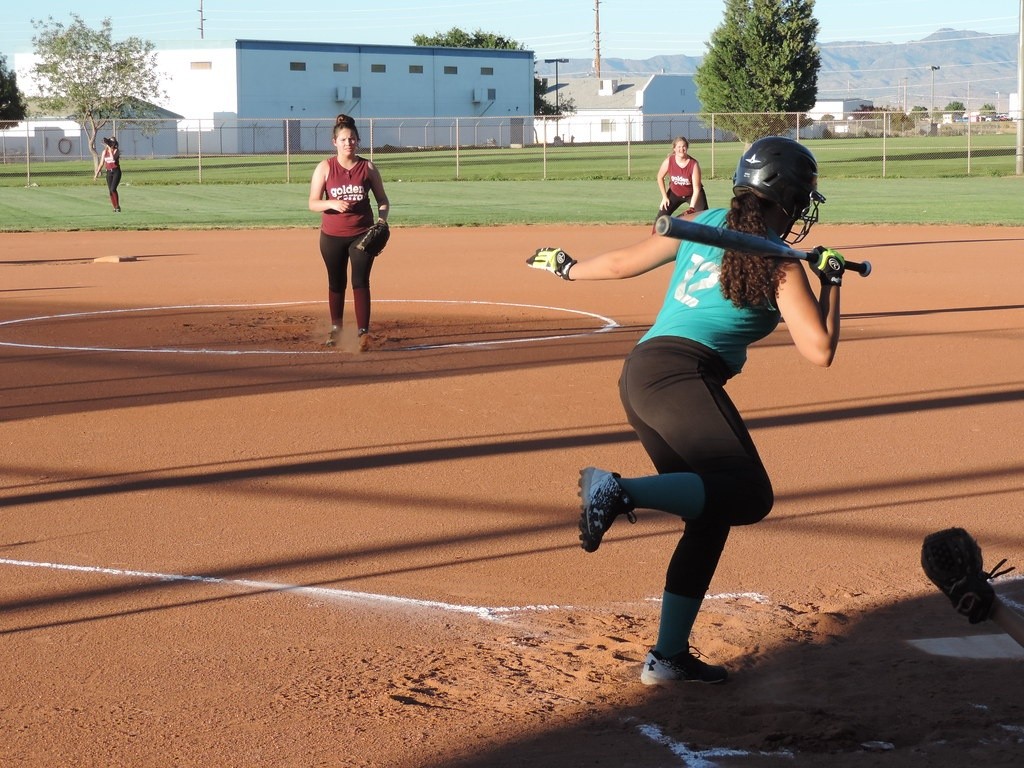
[577,466,639,554]
[640,641,728,686]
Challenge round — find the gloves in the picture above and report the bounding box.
[526,246,576,283]
[808,247,846,287]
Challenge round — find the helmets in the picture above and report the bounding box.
[732,134,821,219]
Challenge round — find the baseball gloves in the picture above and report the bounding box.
[918,527,1000,627]
[356,220,391,257]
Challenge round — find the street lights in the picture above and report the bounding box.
[925,65,941,125]
[545,57,570,149]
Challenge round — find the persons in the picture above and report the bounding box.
[922,528,1023,648]
[308,114,390,351]
[94,136,122,213]
[652,136,709,236]
[525,138,845,685]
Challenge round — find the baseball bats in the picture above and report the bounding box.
[654,213,872,278]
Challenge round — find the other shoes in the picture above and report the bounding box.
[114,207,120,212]
[326,328,340,347]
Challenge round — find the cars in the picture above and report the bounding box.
[995,113,1014,122]
